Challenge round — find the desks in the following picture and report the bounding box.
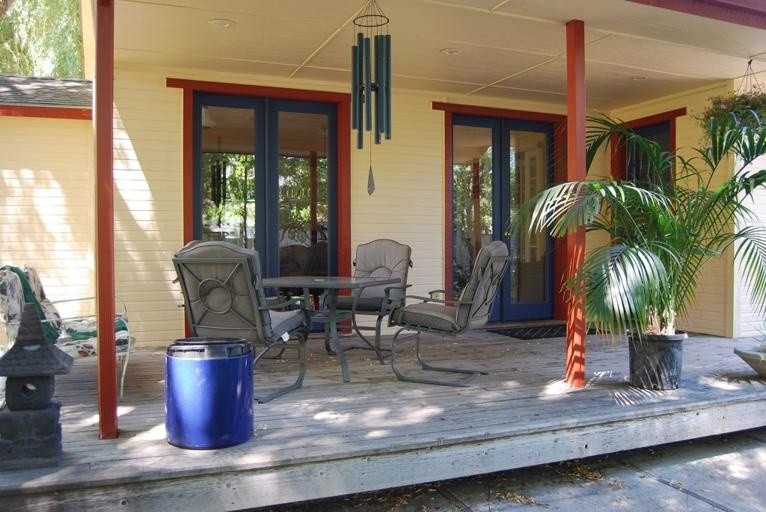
[259,274,401,383]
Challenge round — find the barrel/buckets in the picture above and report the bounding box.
[165,336,255,452]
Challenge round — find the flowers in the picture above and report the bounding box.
[688,83,766,128]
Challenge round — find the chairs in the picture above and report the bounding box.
[0,264,132,402]
[323,239,412,359]
[387,241,509,388]
[172,239,307,403]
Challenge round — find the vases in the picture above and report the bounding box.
[730,345,766,376]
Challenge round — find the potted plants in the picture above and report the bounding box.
[503,102,766,390]
